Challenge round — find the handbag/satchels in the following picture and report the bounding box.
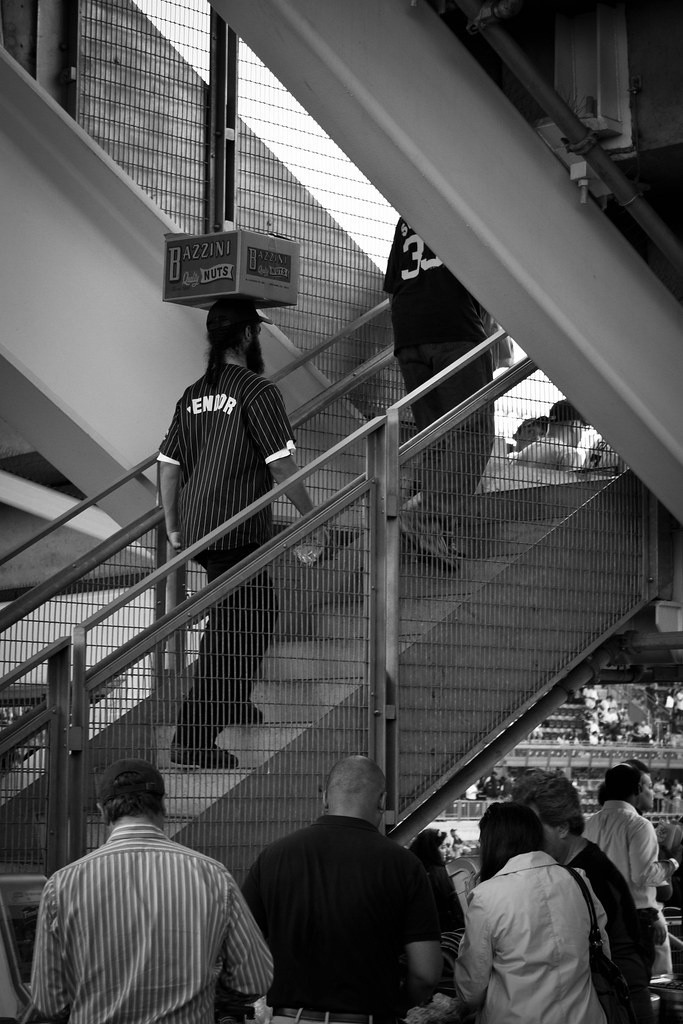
[558,864,638,1024]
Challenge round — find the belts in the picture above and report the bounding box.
[273,1007,395,1024]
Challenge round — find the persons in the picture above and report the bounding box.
[384,215,512,568]
[157,296,328,769]
[31,758,273,1024]
[509,400,624,478]
[408,682,683,1024]
[240,753,447,1024]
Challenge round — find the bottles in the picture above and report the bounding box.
[296,538,322,564]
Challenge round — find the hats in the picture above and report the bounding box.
[206,300,273,332]
[98,758,164,805]
[550,400,590,427]
[513,416,548,438]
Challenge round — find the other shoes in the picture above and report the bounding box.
[170,741,239,768]
[398,493,460,572]
[230,701,262,724]
